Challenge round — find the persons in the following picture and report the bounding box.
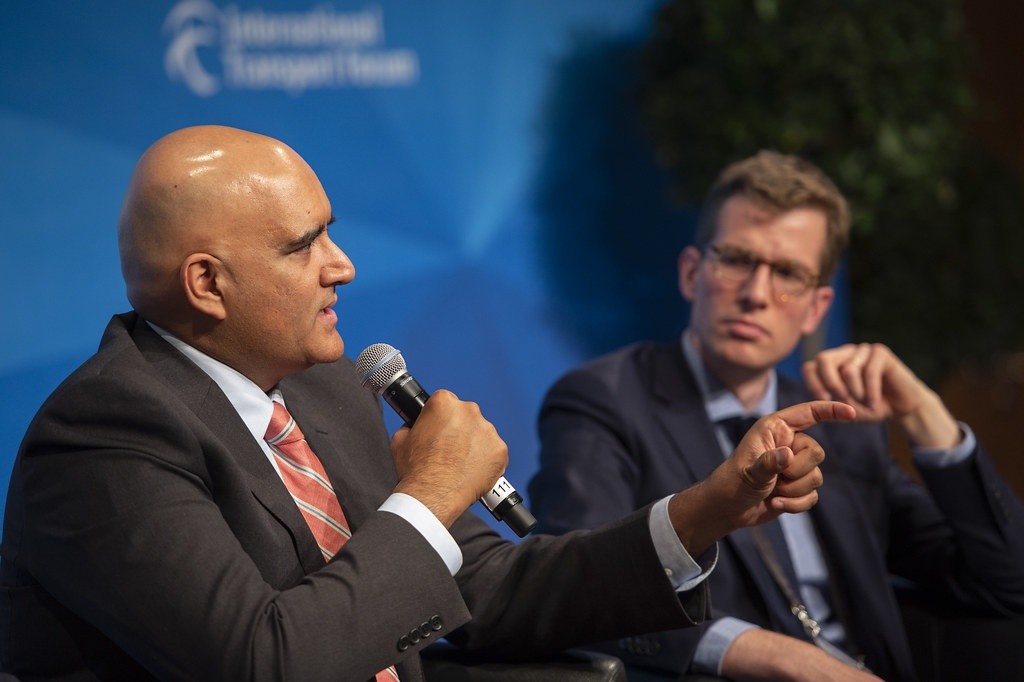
[2,125,856,682]
[529,150,1022,682]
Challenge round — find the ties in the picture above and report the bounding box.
[264,402,400,682]
[719,417,815,639]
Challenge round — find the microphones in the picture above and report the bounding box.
[354,343,539,540]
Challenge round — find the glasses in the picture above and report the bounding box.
[703,242,822,303]
